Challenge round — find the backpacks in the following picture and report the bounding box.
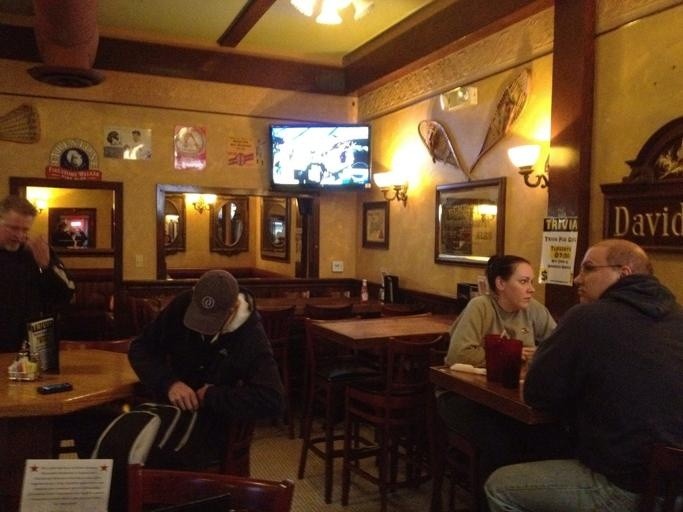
[89,399,204,511]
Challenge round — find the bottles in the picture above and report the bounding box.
[15,351,42,376]
[361,279,369,303]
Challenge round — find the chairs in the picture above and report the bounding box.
[127,460,295,511]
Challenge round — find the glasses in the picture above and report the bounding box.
[579,261,622,276]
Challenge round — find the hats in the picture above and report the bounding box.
[181,269,240,337]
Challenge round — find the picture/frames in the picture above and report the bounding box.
[208,188,249,254]
[48,206,97,257]
[435,178,506,267]
[361,201,390,249]
[163,192,189,253]
[260,195,292,262]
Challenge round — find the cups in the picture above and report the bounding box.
[503,339,523,388]
[484,335,506,382]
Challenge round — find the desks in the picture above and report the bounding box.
[0,347,141,512]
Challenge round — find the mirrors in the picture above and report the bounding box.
[9,176,123,335]
[154,183,319,282]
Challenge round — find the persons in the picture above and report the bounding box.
[435,255,559,485]
[51,221,89,247]
[0,194,78,355]
[123,130,148,160]
[54,269,288,512]
[103,131,124,158]
[483,239,683,511]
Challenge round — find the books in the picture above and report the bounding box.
[26,318,57,372]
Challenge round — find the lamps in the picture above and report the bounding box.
[373,172,406,205]
[289,1,373,25]
[507,146,550,189]
[185,193,217,214]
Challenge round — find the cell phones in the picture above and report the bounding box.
[37,382,72,395]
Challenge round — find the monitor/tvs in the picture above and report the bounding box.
[269,123,372,192]
